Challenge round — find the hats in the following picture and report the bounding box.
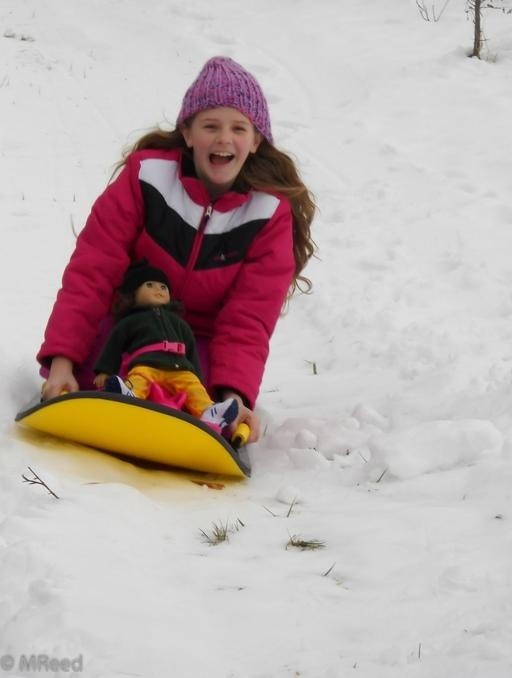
[176,57,273,145]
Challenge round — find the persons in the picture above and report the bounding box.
[36,56,319,445]
[92,257,239,436]
